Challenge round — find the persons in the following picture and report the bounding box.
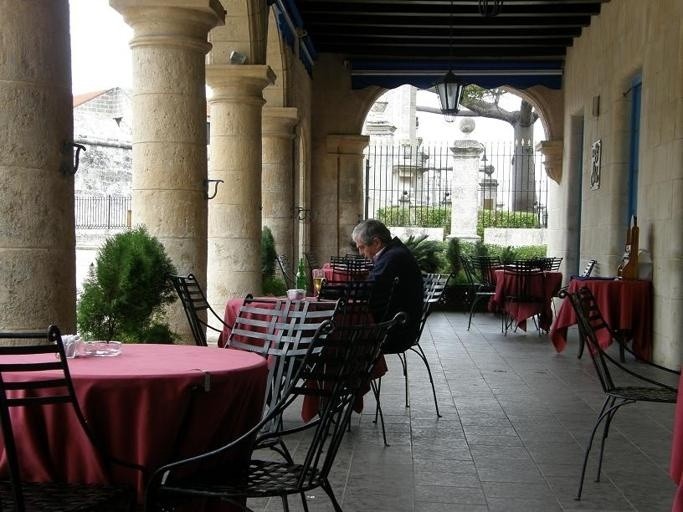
[315,218,425,412]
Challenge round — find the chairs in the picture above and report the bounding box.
[460,254,563,337]
[558,286,678,501]
[0,271,408,512]
[276,252,453,418]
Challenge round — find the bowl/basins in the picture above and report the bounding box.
[285,288,307,300]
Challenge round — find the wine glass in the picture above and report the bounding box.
[312,269,325,298]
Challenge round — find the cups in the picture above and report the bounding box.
[57,342,75,358]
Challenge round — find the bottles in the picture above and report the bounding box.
[296,258,306,289]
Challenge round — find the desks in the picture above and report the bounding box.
[569,276,653,363]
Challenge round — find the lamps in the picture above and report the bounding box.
[430,2,469,113]
